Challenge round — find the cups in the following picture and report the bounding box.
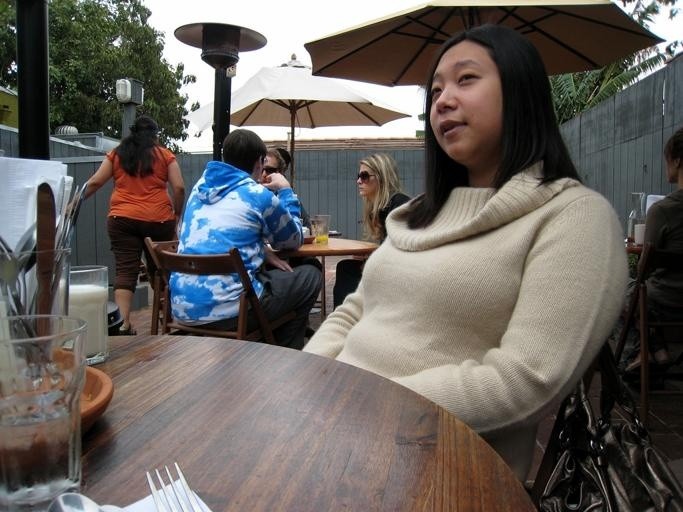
[309,215,330,245]
[0,312,87,509]
[64,264,109,364]
[634,224,646,245]
[1,250,69,317]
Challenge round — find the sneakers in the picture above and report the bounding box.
[626,349,668,371]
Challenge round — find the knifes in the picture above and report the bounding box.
[35,175,88,352]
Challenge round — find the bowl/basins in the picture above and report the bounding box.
[303,236,315,244]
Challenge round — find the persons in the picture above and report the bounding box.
[256,146,325,340]
[276,147,291,178]
[298,22,630,487]
[618,125,681,384]
[166,127,323,351]
[61,114,186,336]
[330,151,413,314]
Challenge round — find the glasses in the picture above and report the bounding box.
[358,171,375,184]
[262,167,278,174]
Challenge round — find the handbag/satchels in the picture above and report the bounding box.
[539,403,683,512]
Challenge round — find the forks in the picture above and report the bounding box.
[145,461,200,512]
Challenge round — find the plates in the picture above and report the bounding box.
[0,361,114,470]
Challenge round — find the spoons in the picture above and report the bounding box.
[12,222,38,314]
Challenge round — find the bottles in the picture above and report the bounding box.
[628,192,645,242]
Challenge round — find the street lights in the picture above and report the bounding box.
[114,77,145,139]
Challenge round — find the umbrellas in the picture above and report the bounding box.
[182,52,418,194]
[299,7,672,87]
